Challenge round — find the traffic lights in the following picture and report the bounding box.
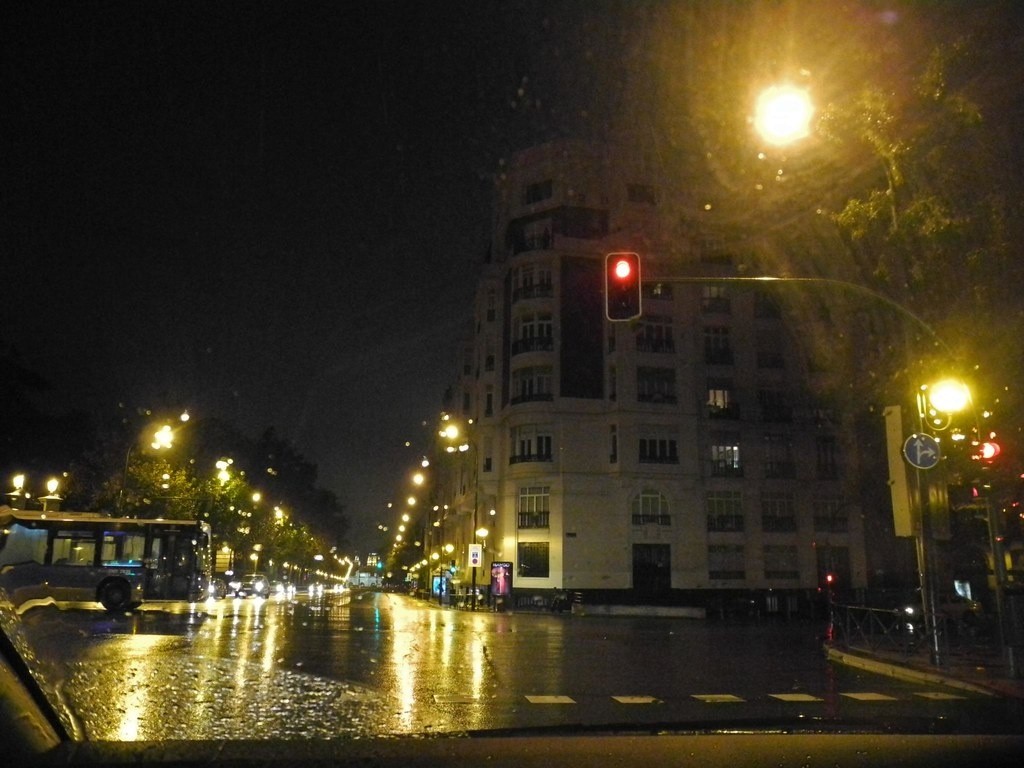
[605,251,643,319]
[921,372,951,430]
[975,438,1004,498]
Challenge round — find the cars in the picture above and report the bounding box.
[309,584,323,592]
[334,583,343,589]
[270,580,284,594]
[284,582,297,593]
[236,575,271,598]
[209,579,227,599]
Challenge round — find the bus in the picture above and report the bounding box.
[0,507,213,614]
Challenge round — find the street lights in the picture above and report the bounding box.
[753,84,948,666]
[393,459,443,606]
[118,427,173,510]
[446,424,477,612]
